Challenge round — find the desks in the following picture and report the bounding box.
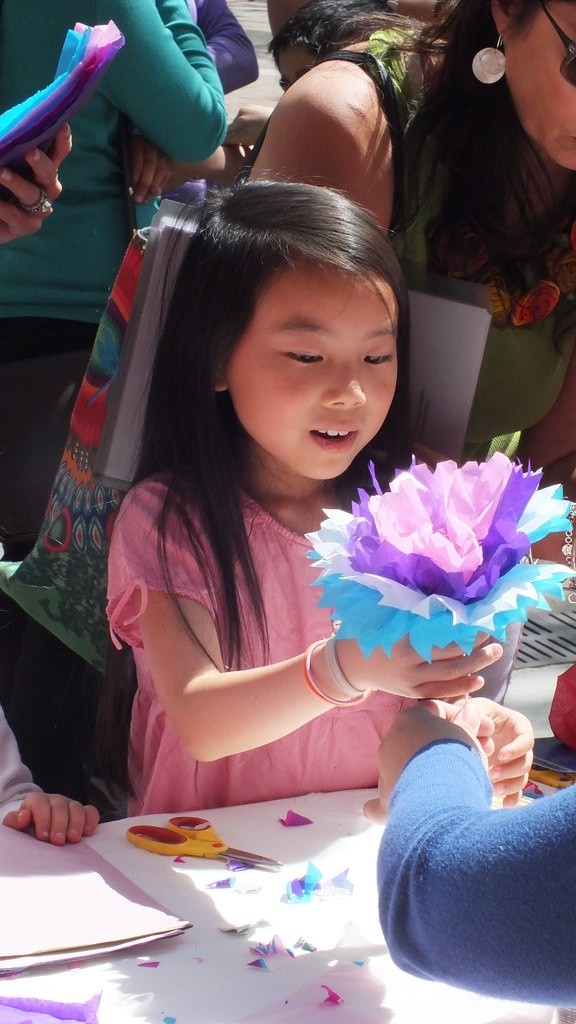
[0,788,576,1024]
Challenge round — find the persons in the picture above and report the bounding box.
[0,0,576,844]
[363,701,576,1024]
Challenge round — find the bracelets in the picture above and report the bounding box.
[304,642,372,706]
[306,639,369,705]
[326,636,367,698]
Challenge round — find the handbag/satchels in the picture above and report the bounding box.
[0,197,199,821]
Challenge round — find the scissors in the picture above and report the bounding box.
[126,814,284,874]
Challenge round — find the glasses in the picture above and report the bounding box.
[277,66,312,89]
[540,1,576,87]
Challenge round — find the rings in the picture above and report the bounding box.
[18,186,52,214]
